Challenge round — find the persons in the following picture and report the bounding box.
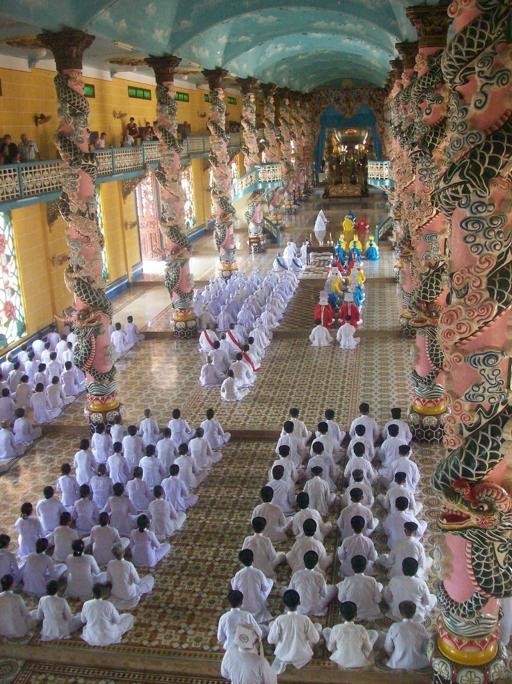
[1,116,157,166]
[1,316,145,471]
[309,209,382,350]
[1,408,230,647]
[219,403,437,684]
[190,239,313,403]
